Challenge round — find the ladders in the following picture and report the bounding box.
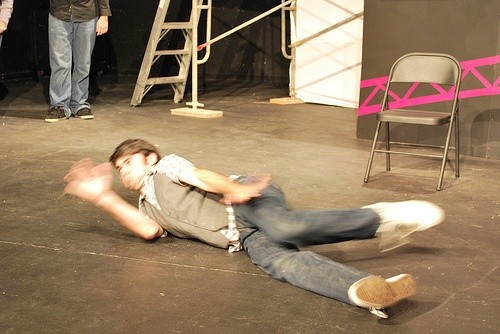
[131,0,203,107]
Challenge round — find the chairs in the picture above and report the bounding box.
[364,52,463,191]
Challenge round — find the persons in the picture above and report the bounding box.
[64,139,446,319]
[0,0,15,46]
[44,0,111,121]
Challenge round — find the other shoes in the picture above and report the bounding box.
[347,272,417,318]
[361,199,445,237]
[44,106,67,122]
[75,107,94,119]
